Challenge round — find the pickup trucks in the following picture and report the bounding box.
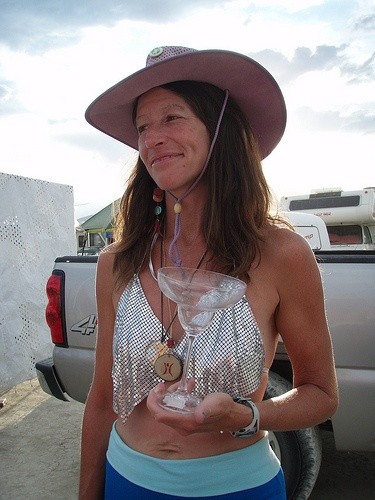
[35,250,375,500]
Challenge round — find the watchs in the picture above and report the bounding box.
[230,395,260,438]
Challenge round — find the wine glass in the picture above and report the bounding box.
[154,268,247,414]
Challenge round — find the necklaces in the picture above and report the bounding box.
[149,186,216,382]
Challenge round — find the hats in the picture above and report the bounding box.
[85,45,287,160]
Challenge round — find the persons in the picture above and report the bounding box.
[79,45,340,500]
